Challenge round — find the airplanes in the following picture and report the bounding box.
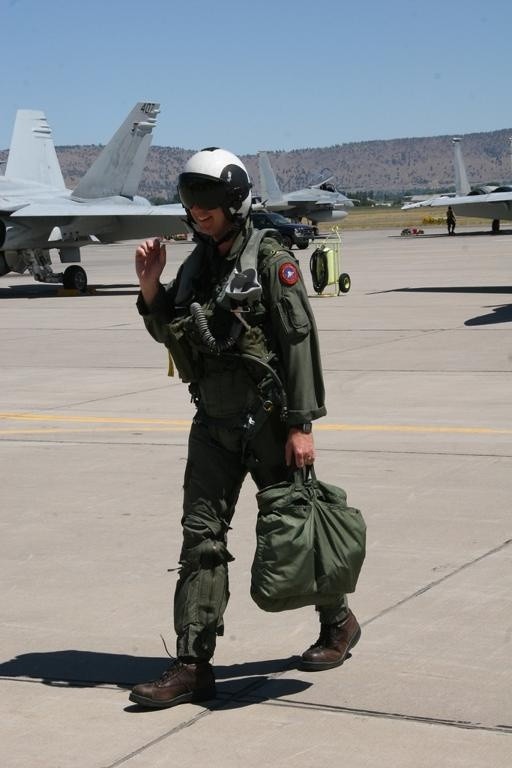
[0,101,265,296]
[250,149,359,238]
[399,137,511,234]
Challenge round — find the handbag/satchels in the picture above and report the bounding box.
[250,479,366,612]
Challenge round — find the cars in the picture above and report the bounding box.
[249,212,314,250]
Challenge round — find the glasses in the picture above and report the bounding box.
[176,182,252,211]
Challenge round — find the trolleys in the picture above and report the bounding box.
[312,224,351,296]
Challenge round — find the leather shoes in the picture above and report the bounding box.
[129,658,216,710]
[300,608,362,671]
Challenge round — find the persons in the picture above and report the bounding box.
[129,147,361,708]
[447,205,457,235]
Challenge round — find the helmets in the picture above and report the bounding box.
[178,146,253,226]
